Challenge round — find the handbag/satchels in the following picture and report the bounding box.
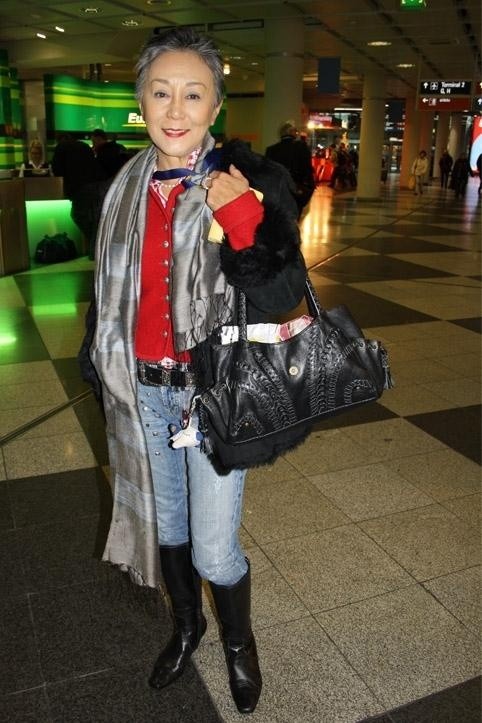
[291,168,314,207]
[408,175,415,189]
[199,275,397,477]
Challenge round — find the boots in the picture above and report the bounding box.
[148,541,208,691]
[208,555,263,714]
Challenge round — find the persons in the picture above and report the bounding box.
[52,129,129,263]
[231,121,316,223]
[73,26,309,716]
[476,153,482,196]
[128,148,140,158]
[19,139,54,178]
[412,150,428,195]
[316,143,358,190]
[450,152,475,198]
[438,149,453,190]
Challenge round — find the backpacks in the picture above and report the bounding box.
[334,150,346,165]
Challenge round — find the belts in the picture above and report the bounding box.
[137,361,198,387]
